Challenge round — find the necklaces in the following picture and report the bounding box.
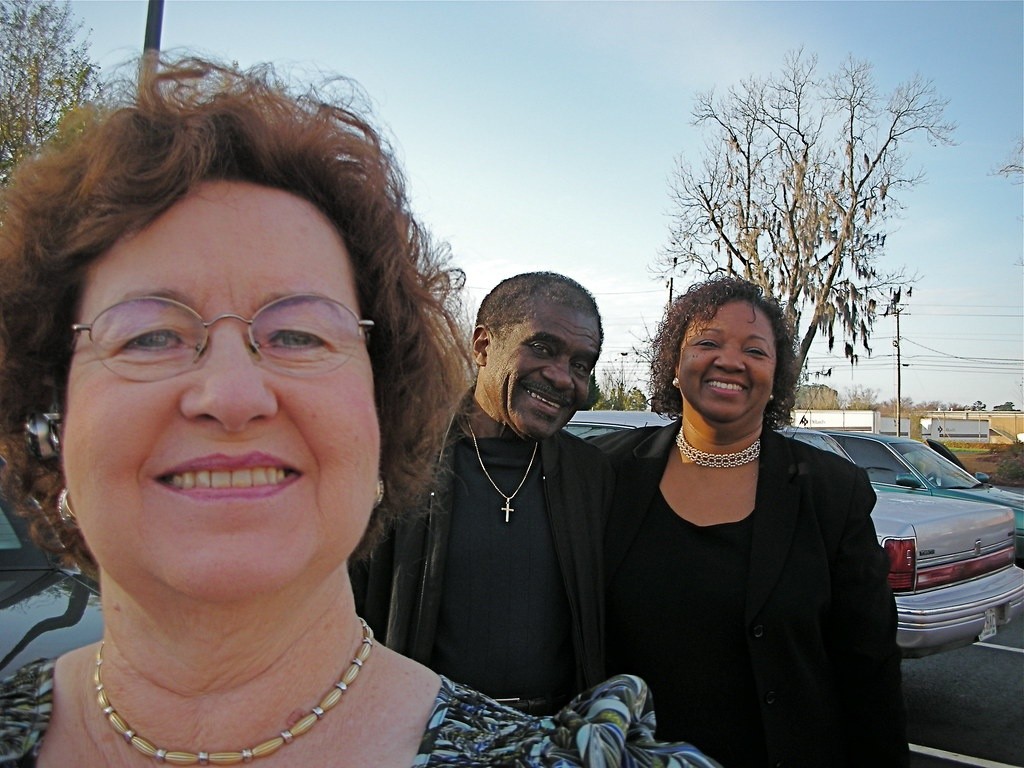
[94,617,373,767]
[466,416,537,522]
[676,426,761,468]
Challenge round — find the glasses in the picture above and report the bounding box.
[66,294,375,383]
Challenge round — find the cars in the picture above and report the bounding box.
[561,407,679,443]
[816,429,1024,566]
[777,424,1024,660]
[0,499,107,686]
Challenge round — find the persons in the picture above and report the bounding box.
[0,52,717,768]
[349,272,615,716]
[581,281,911,767]
